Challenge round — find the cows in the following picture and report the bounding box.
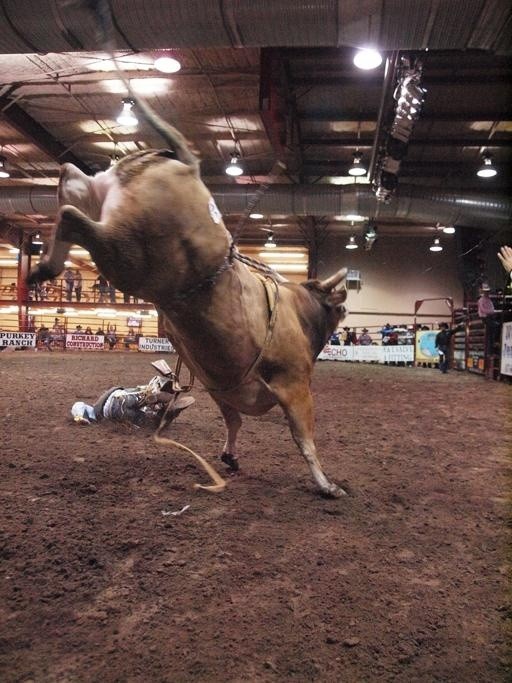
[25,37,351,499]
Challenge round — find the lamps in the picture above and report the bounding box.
[224,152,244,177]
[366,49,430,203]
[116,97,139,126]
[430,235,442,251]
[264,234,277,249]
[346,229,359,250]
[346,148,368,177]
[473,156,498,180]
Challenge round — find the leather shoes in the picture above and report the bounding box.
[165,396,195,411]
[145,391,172,404]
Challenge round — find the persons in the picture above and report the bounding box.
[0,266,154,352]
[71,379,195,428]
[495,244,512,281]
[322,280,511,370]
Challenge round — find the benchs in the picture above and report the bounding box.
[1,278,159,349]
